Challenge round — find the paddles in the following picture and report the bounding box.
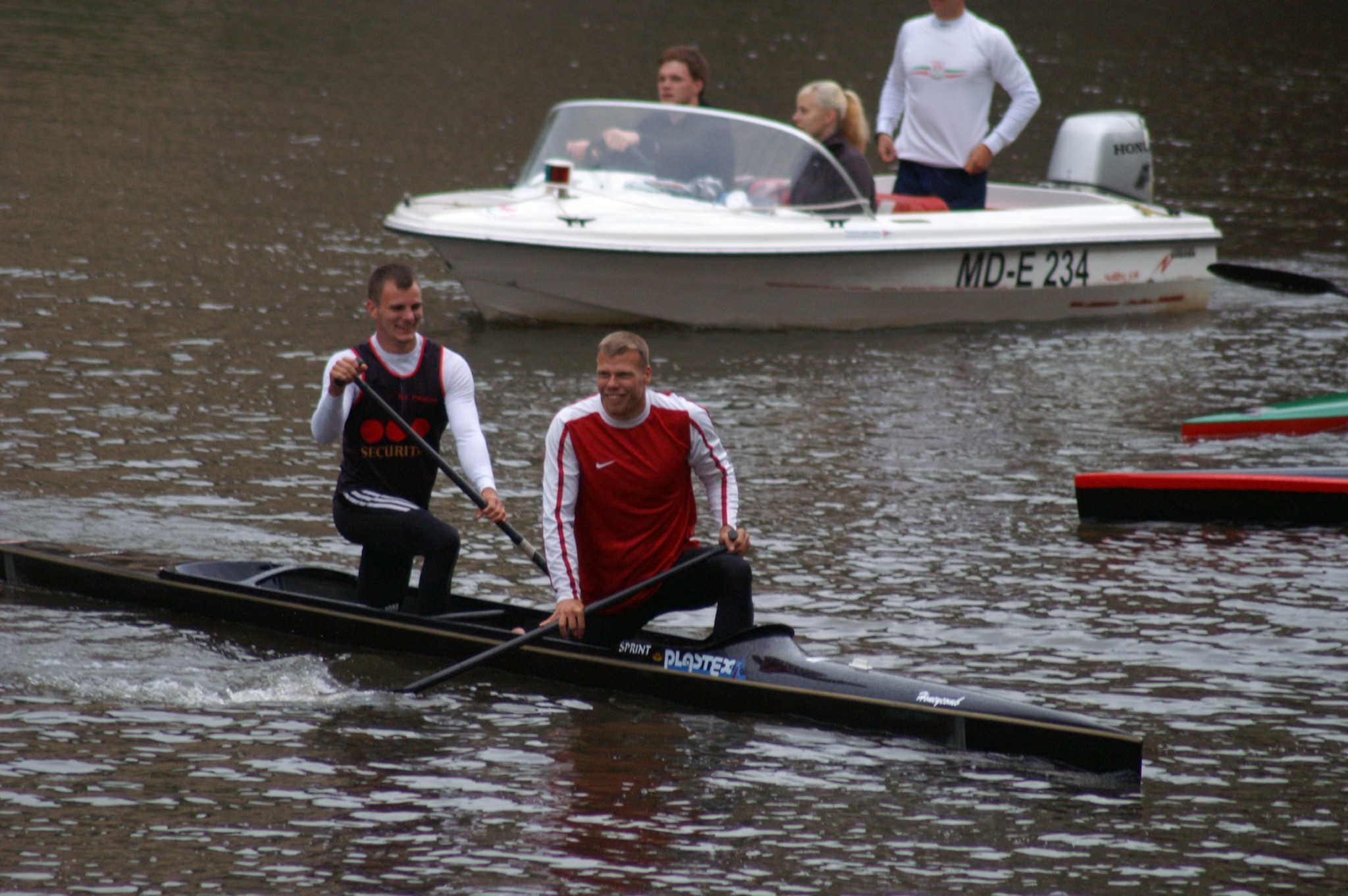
[1205,262,1348,298]
[386,530,739,694]
[335,358,551,577]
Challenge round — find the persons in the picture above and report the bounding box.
[567,45,734,200]
[875,1,1042,211]
[310,264,508,615]
[788,80,878,215]
[511,332,754,642]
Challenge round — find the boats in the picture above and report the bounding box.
[1073,463,1348,524]
[0,526,1144,774]
[1182,393,1348,441]
[382,101,1222,333]
